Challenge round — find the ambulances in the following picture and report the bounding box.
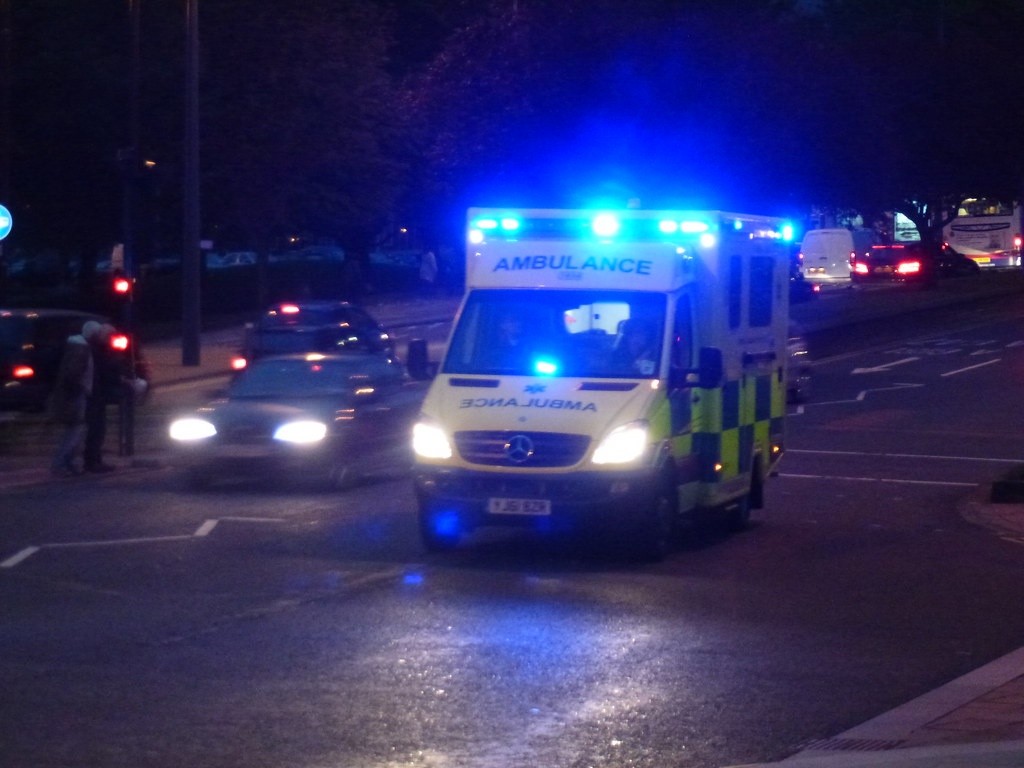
[407,206,792,558]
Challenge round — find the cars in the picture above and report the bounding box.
[241,301,396,357]
[0,310,152,413]
[170,352,433,492]
[848,240,981,286]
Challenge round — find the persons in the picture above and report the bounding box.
[416,251,438,300]
[46,319,148,479]
[483,309,660,376]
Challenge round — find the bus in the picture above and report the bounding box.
[940,188,1024,269]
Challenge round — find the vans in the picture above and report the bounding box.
[799,229,884,288]
